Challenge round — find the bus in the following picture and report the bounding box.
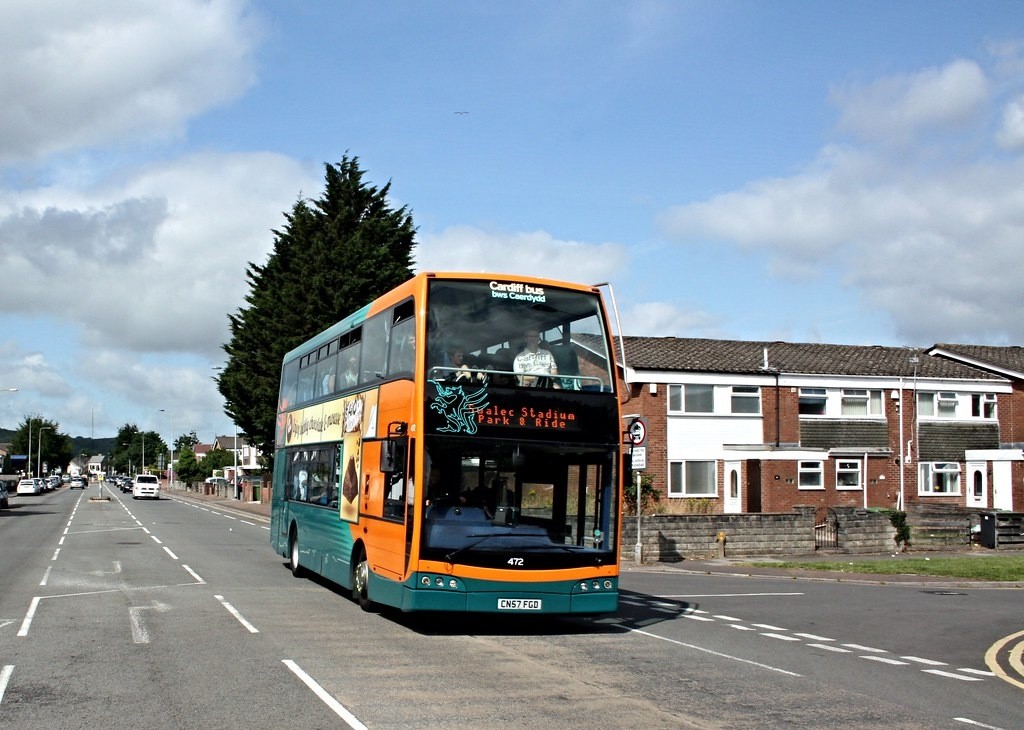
[269,270,636,612]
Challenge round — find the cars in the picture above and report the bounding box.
[16,478,41,495]
[203,473,265,488]
[0,481,10,510]
[33,472,133,495]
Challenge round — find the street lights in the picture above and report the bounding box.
[891,379,908,509]
[158,409,175,491]
[134,431,145,475]
[122,442,131,477]
[37,426,54,476]
[27,417,44,479]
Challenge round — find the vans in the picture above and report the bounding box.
[131,474,162,501]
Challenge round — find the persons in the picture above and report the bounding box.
[443,344,484,380]
[322,329,415,395]
[298,470,514,519]
[513,328,561,389]
